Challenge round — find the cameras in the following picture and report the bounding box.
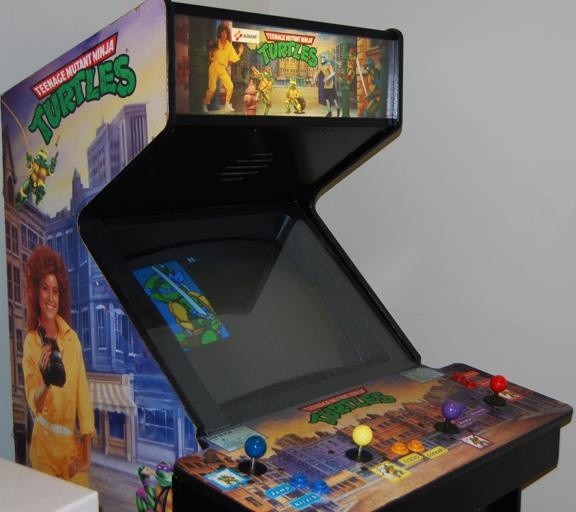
[38,326,66,388]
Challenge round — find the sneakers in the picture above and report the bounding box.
[224,102,236,112]
[201,103,209,112]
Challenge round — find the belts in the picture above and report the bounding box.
[35,415,76,435]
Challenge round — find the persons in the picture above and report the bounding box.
[19,243,97,489]
[200,23,247,112]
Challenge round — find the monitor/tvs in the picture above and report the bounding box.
[102,201,419,429]
